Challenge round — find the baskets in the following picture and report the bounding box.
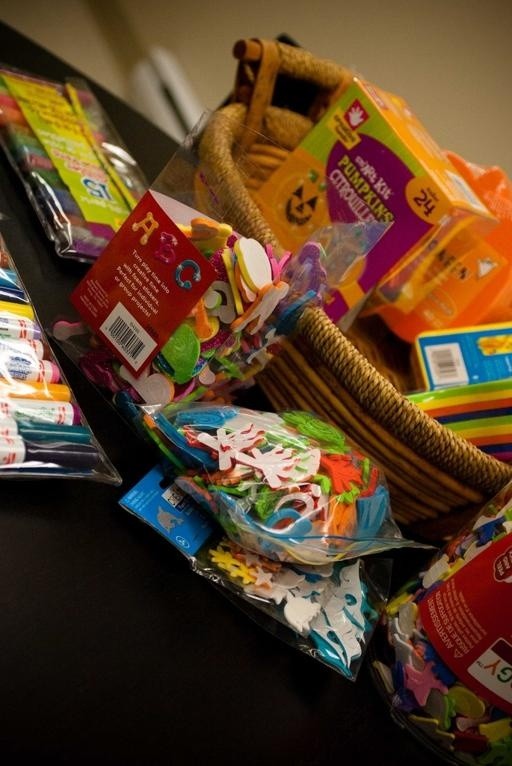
[196,38,511,522]
